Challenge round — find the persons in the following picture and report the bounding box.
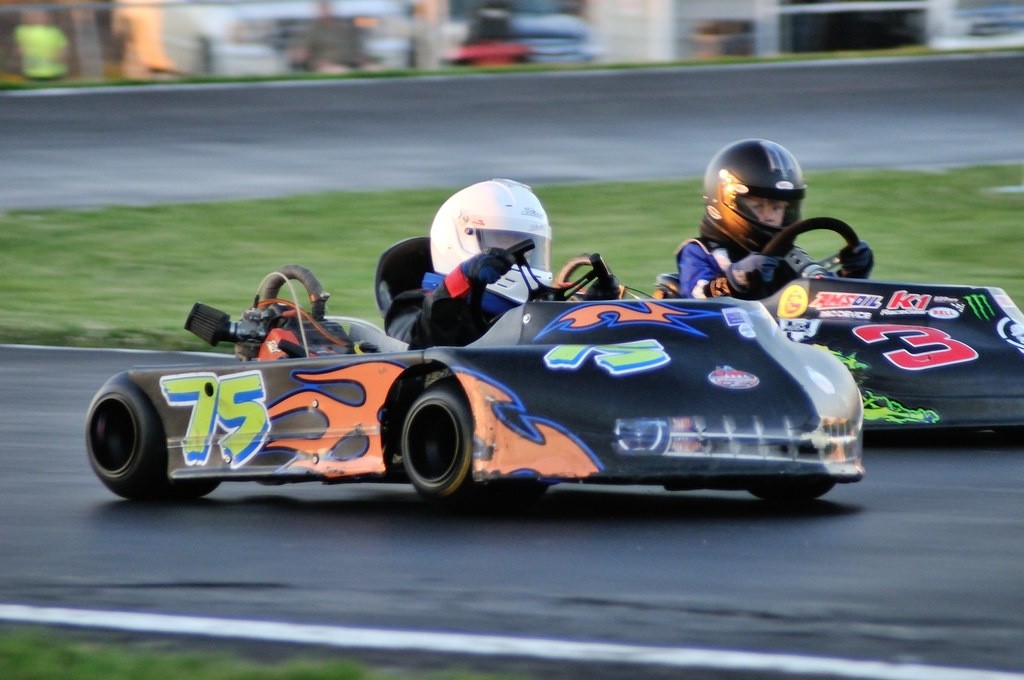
[11,5,71,81]
[666,139,873,304]
[286,0,367,73]
[453,0,534,68]
[378,179,617,350]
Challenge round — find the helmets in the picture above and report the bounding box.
[430,178,554,305]
[700,139,806,255]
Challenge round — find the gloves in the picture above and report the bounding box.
[727,252,780,296]
[584,275,619,302]
[460,248,515,282]
[838,242,874,280]
